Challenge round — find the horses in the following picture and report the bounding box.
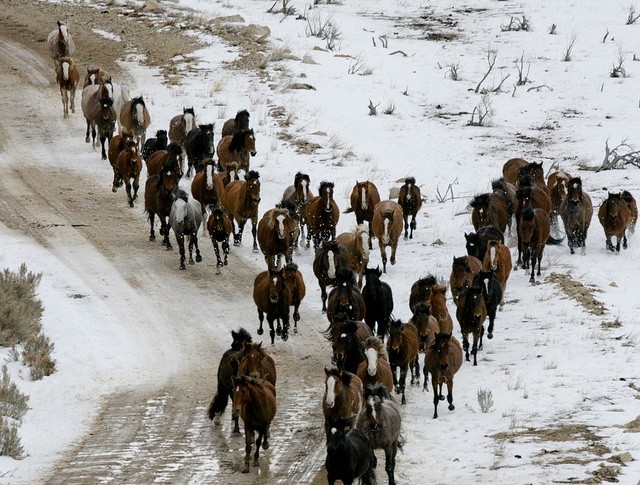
[143,167,182,251]
[503,157,544,188]
[469,192,510,233]
[97,99,117,159]
[483,240,512,311]
[232,343,276,438]
[398,177,422,241]
[464,225,504,263]
[169,107,198,174]
[386,320,420,403]
[450,256,482,304]
[82,76,113,148]
[284,264,306,333]
[119,96,151,152]
[599,193,630,252]
[327,269,366,323]
[515,169,553,218]
[171,188,207,271]
[322,367,363,422]
[473,270,502,338]
[276,171,314,250]
[409,303,441,354]
[219,162,247,188]
[456,286,487,365]
[207,204,233,267]
[253,263,292,344]
[208,330,254,424]
[191,159,224,238]
[343,179,381,249]
[300,182,339,253]
[223,171,261,253]
[422,332,462,419]
[362,266,393,343]
[373,201,404,273]
[258,209,299,272]
[313,241,349,313]
[217,129,257,171]
[334,225,371,276]
[143,130,168,162]
[146,143,183,176]
[117,141,143,207]
[357,382,406,485]
[56,57,80,119]
[548,172,573,213]
[410,277,453,335]
[319,313,374,373]
[109,131,133,192]
[493,179,519,211]
[231,373,277,473]
[47,20,75,72]
[560,178,593,254]
[83,68,111,88]
[185,123,215,179]
[357,337,393,393]
[325,411,377,485]
[222,110,250,135]
[517,208,564,282]
[622,190,637,233]
